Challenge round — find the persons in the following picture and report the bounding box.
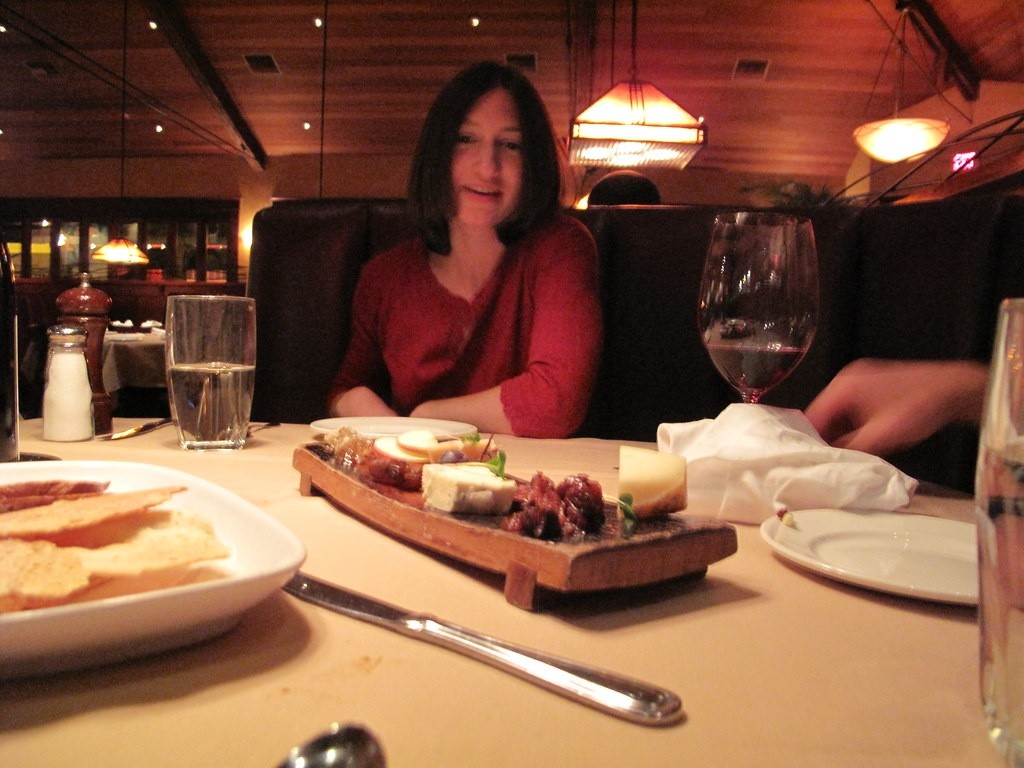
[802,357,989,457]
[324,60,607,439]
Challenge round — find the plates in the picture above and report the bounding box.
[309,416,476,441]
[759,509,977,605]
[292,443,739,611]
[0,463,306,684]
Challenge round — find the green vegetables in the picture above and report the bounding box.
[455,432,507,478]
[603,493,644,537]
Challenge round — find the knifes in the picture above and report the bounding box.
[282,572,685,726]
[97,418,171,440]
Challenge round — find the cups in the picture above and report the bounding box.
[976,298,1024,765]
[165,295,256,452]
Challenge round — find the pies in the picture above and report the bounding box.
[0,479,231,617]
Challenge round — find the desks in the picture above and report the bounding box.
[20,321,167,398]
[1,419,1024,768]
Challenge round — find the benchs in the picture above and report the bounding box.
[248,202,1022,488]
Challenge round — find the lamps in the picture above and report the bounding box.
[563,0,706,171]
[851,7,953,166]
[93,1,149,264]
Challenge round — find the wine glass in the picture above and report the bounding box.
[699,211,820,403]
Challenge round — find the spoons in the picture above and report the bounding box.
[275,725,387,768]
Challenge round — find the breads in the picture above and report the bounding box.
[618,445,688,517]
[420,463,516,516]
[368,431,438,489]
[427,437,496,463]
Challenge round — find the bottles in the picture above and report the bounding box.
[0,230,18,462]
[43,325,94,442]
[56,271,114,433]
